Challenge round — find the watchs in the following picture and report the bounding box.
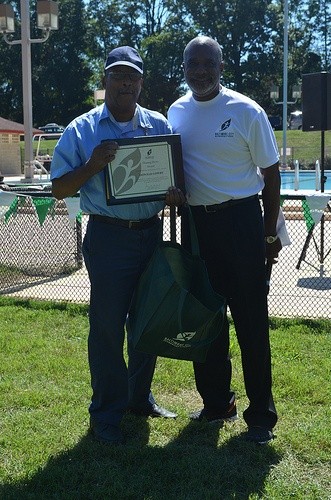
[263,234,278,246]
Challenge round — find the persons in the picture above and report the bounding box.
[48,46,185,445]
[165,34,284,445]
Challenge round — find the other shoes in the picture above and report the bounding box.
[127,403,179,418]
[89,417,119,442]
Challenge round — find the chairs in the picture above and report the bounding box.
[296,199,331,270]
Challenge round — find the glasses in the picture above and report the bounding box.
[105,71,143,82]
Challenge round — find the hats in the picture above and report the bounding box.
[104,45,144,74]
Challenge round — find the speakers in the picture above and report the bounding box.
[302,72,331,132]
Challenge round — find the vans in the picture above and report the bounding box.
[267,111,303,131]
[38,126,66,140]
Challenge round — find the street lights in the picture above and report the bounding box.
[0,0,59,181]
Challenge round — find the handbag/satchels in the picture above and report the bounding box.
[127,190,227,365]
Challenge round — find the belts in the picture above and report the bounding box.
[90,213,160,231]
[189,194,259,214]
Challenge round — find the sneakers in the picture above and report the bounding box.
[246,428,273,445]
[189,408,238,423]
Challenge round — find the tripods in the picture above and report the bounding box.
[295,130,331,275]
